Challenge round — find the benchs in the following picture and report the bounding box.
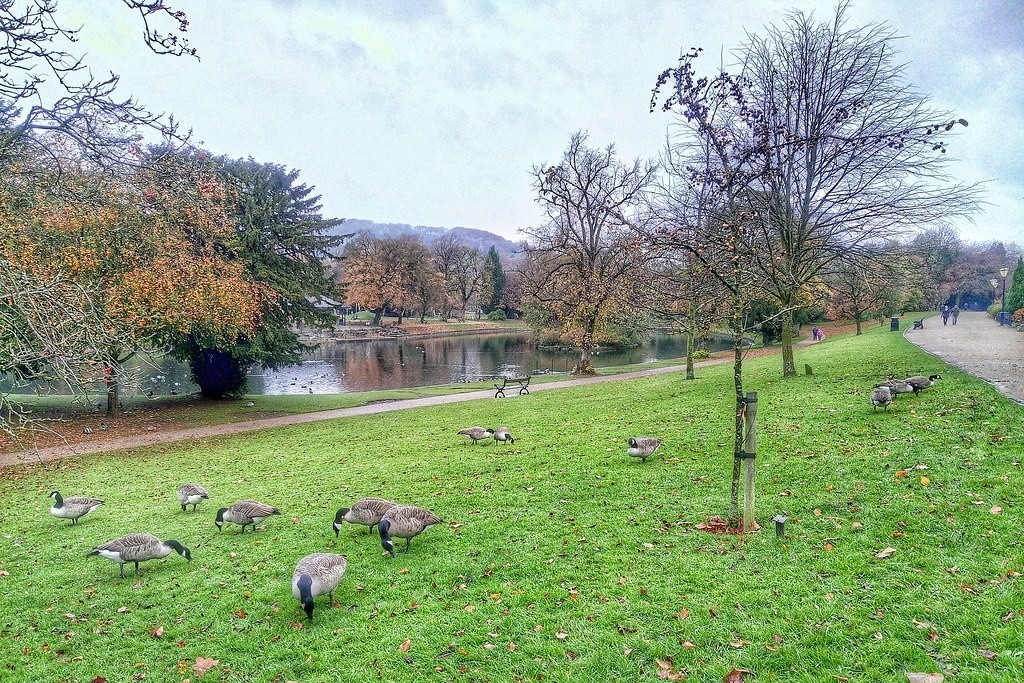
[913,317,924,329]
[494,376,530,398]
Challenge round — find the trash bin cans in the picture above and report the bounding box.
[890,317,900,331]
[940,305,945,312]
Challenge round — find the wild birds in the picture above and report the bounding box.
[316,370,346,377]
[46,491,106,526]
[628,436,661,463]
[177,483,210,512]
[146,390,154,397]
[396,361,406,366]
[150,373,187,387]
[89,404,102,414]
[291,552,350,620]
[416,346,425,353]
[457,426,515,446]
[170,388,178,395]
[332,498,449,557]
[876,374,945,399]
[244,400,255,407]
[215,500,283,536]
[83,426,93,434]
[100,422,108,431]
[871,385,893,411]
[83,533,194,579]
[290,377,313,393]
[118,402,123,411]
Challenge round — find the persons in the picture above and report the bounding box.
[962,302,969,311]
[816,328,823,341]
[941,306,950,326]
[812,325,818,341]
[951,304,960,325]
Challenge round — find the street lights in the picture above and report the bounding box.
[998,268,1010,325]
[989,278,999,305]
